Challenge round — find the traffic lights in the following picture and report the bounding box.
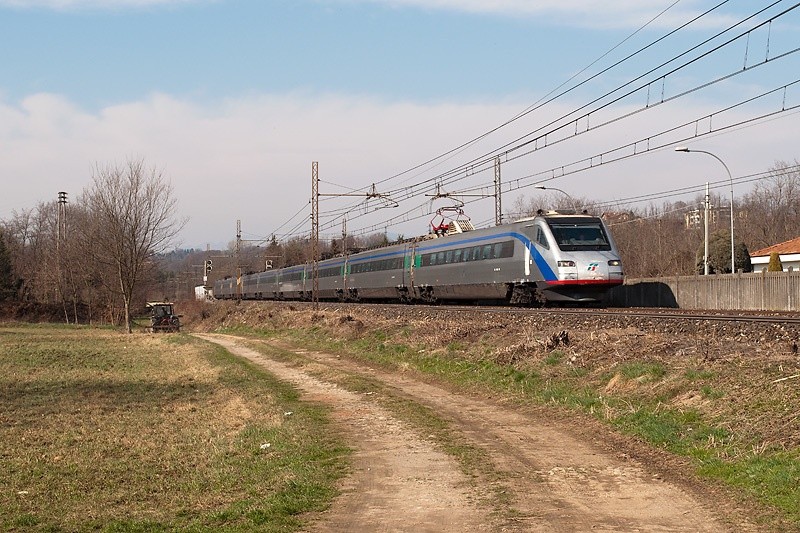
[266,260,273,271]
[207,260,212,271]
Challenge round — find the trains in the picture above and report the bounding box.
[213,211,625,307]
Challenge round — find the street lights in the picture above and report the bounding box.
[535,186,577,214]
[675,147,734,275]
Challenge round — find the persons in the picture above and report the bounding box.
[161,307,167,316]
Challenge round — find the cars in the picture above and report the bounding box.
[151,302,179,332]
